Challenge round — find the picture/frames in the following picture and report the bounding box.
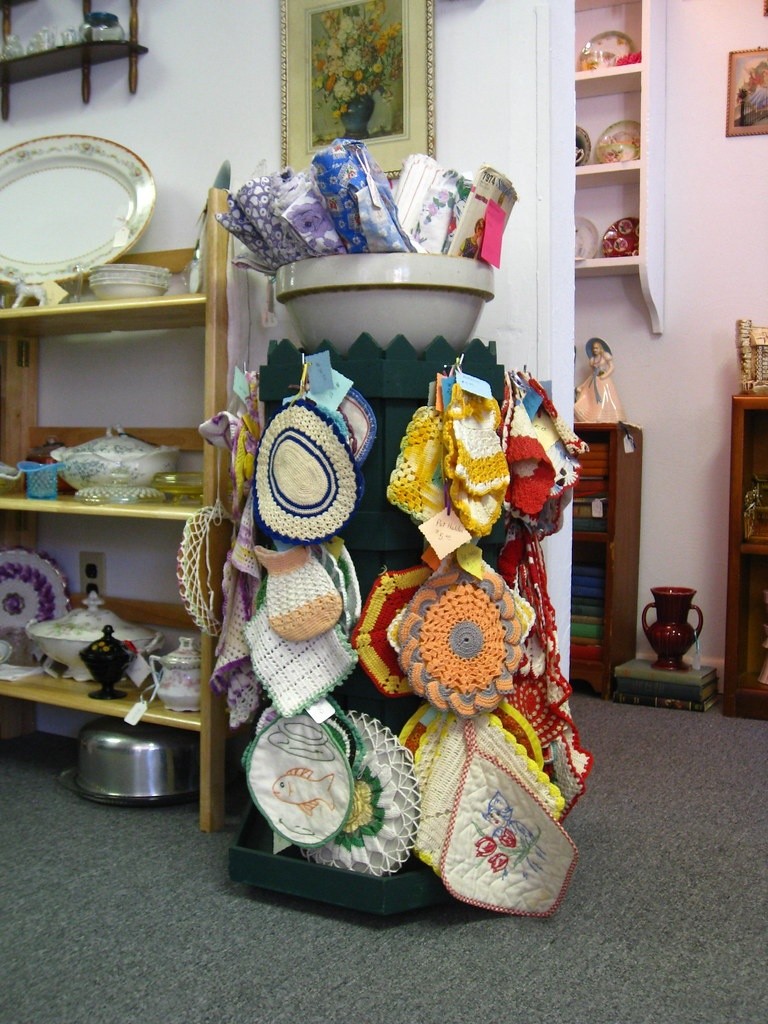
[726,48,768,136]
[279,0,437,179]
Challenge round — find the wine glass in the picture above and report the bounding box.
[79,624,134,699]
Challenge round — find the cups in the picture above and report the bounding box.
[149,636,200,712]
[0,624,32,666]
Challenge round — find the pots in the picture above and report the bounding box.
[58,716,199,806]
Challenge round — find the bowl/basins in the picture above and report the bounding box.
[88,262,171,297]
[273,252,495,347]
[24,589,162,681]
[24,423,204,503]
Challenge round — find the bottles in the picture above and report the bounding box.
[4,11,125,60]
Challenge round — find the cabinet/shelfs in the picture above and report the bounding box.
[575,0,666,334]
[570,421,643,702]
[0,189,233,838]
[723,395,768,716]
[0,0,149,120]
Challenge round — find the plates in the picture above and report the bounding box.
[0,548,70,664]
[571,444,607,661]
[579,30,638,71]
[595,119,640,164]
[602,216,638,257]
[576,126,591,166]
[0,136,156,285]
[575,215,599,259]
